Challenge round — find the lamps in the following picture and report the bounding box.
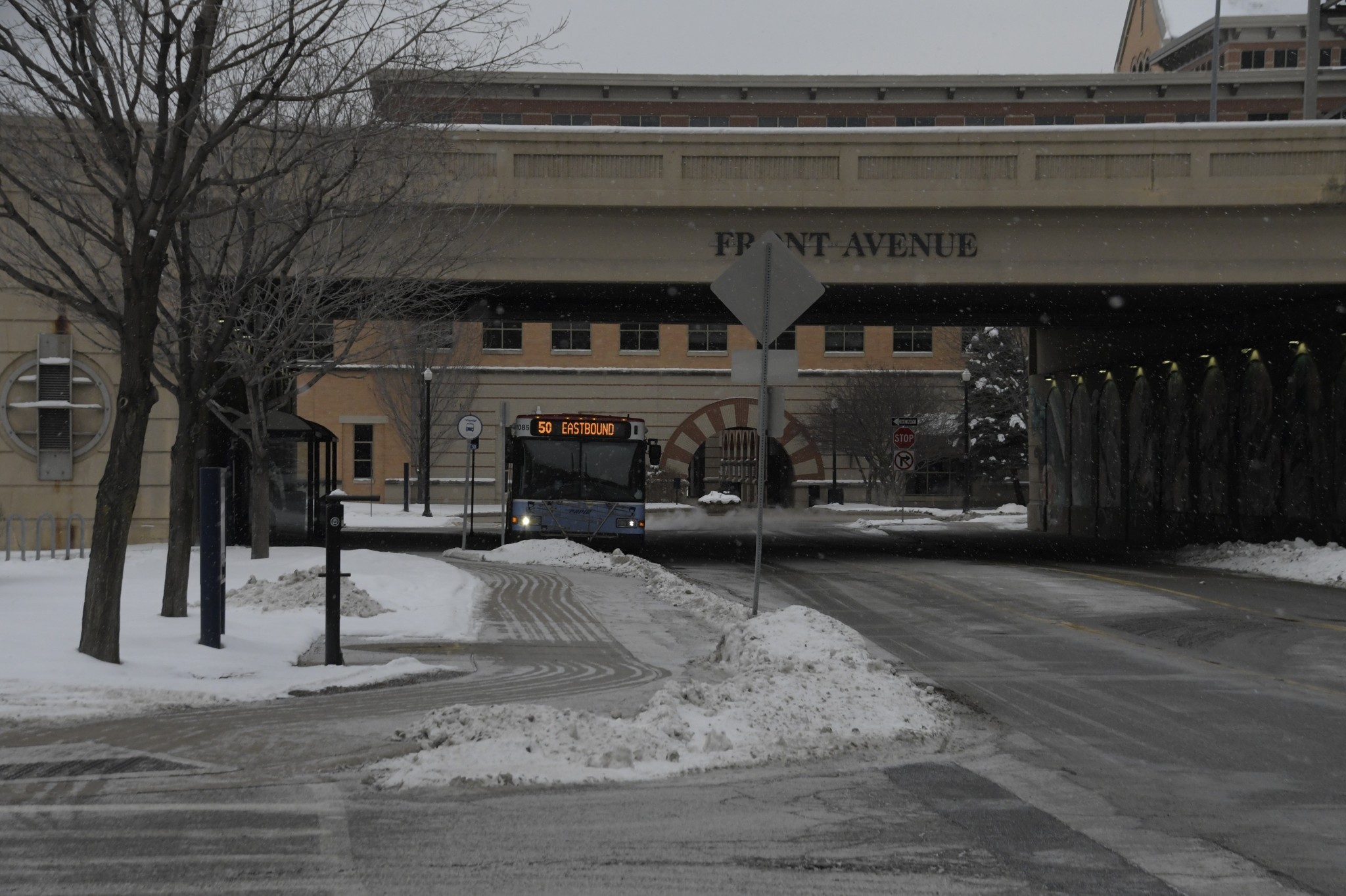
[1044,377,1052,381]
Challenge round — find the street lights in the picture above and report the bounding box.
[962,368,971,515]
[830,398,839,503]
[423,367,432,516]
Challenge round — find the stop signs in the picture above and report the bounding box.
[893,427,916,450]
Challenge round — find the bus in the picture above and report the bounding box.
[506,411,662,555]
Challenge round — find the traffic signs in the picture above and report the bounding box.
[891,417,918,426]
